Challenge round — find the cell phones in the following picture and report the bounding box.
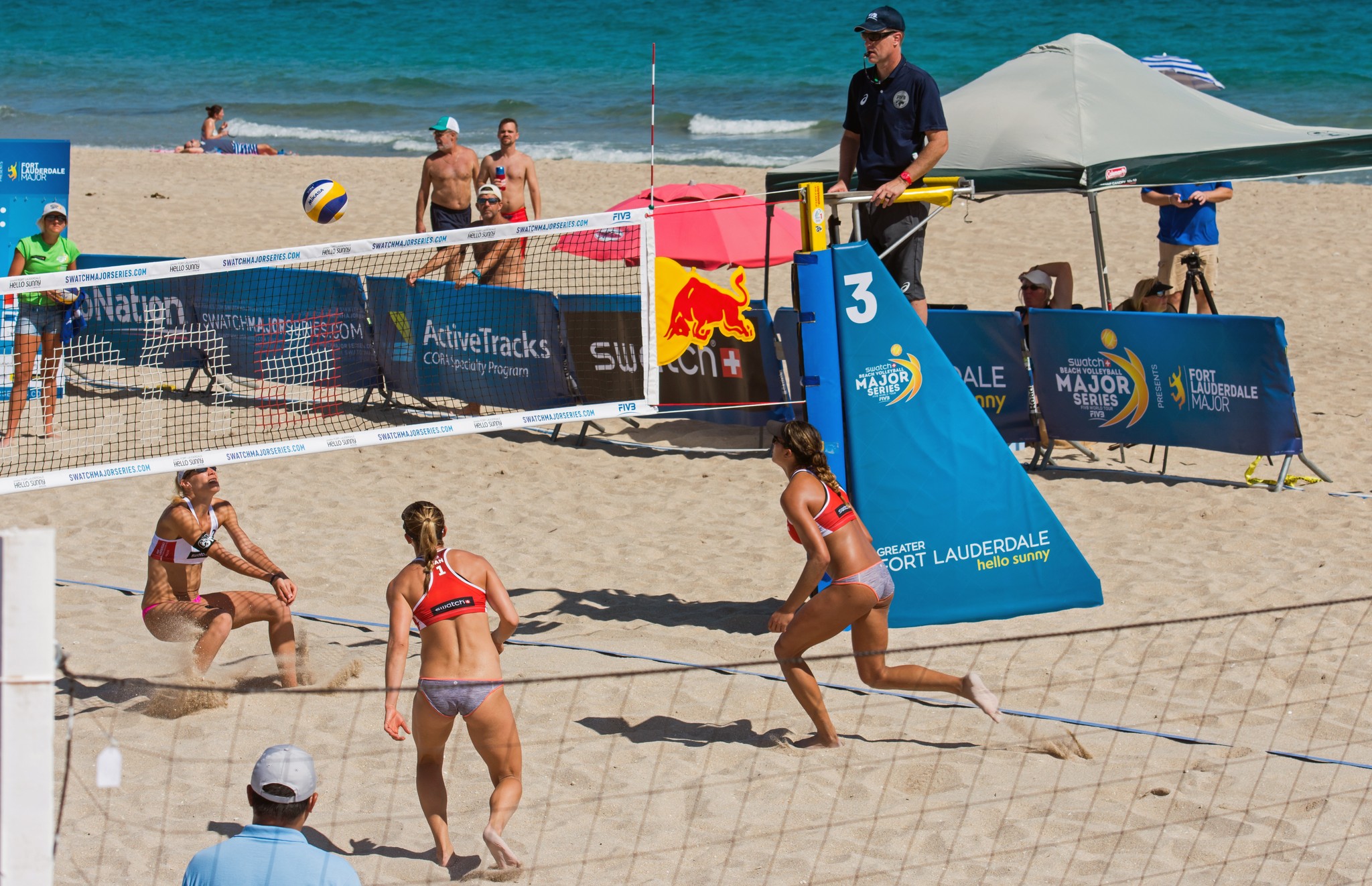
[1182,200,1192,203]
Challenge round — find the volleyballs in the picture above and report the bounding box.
[302,179,348,224]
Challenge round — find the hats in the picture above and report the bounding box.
[477,184,502,202]
[854,5,906,32]
[250,744,315,805]
[42,202,68,217]
[1021,284,1042,291]
[429,116,460,134]
[178,448,213,489]
[1018,270,1052,290]
[1144,281,1174,297]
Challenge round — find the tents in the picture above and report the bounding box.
[763,32,1372,460]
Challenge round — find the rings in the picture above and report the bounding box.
[885,196,890,199]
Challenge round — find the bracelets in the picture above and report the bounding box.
[1034,265,1038,271]
[219,127,223,130]
[1167,193,1174,206]
[269,574,278,584]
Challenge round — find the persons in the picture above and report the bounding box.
[140,463,311,691]
[767,420,1002,747]
[406,184,526,290]
[0,202,80,447]
[1015,262,1073,416]
[414,117,481,282]
[1141,181,1233,316]
[476,118,541,263]
[827,5,949,330]
[175,104,299,156]
[384,500,525,879]
[183,744,363,886]
[1111,278,1179,313]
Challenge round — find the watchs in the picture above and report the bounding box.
[472,269,481,281]
[900,171,913,186]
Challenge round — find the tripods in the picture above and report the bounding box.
[1179,271,1219,315]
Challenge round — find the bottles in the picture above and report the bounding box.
[495,166,506,191]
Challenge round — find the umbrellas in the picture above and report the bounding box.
[1136,52,1224,94]
[551,180,803,271]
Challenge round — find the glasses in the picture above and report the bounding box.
[46,214,65,222]
[190,140,193,147]
[1155,290,1170,297]
[861,30,899,41]
[182,466,217,480]
[476,198,500,205]
